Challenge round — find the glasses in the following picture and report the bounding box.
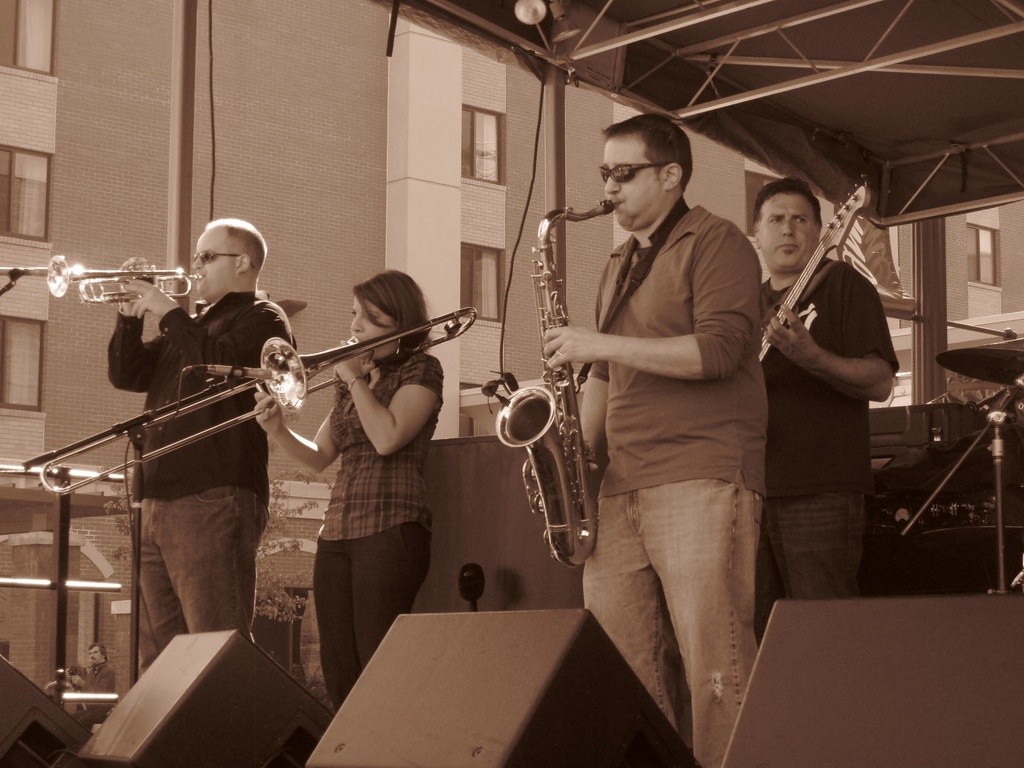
[193,250,255,269]
[600,163,669,183]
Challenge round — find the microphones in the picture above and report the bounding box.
[184,364,273,379]
[0,267,49,276]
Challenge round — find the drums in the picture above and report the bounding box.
[864,423,1024,592]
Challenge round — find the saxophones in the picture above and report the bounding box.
[494,199,623,565]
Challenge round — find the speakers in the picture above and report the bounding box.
[0,595,1024,768]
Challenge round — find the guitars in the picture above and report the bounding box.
[760,174,873,362]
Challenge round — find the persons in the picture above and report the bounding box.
[108,219,294,672]
[757,177,899,637]
[72,643,116,730]
[522,116,765,768]
[253,271,443,713]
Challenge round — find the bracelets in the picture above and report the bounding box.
[348,376,365,392]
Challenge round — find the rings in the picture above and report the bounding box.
[138,299,141,303]
[557,350,563,356]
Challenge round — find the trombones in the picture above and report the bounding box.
[39,306,480,497]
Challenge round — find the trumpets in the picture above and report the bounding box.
[47,255,204,305]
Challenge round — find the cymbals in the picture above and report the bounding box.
[934,347,1024,386]
